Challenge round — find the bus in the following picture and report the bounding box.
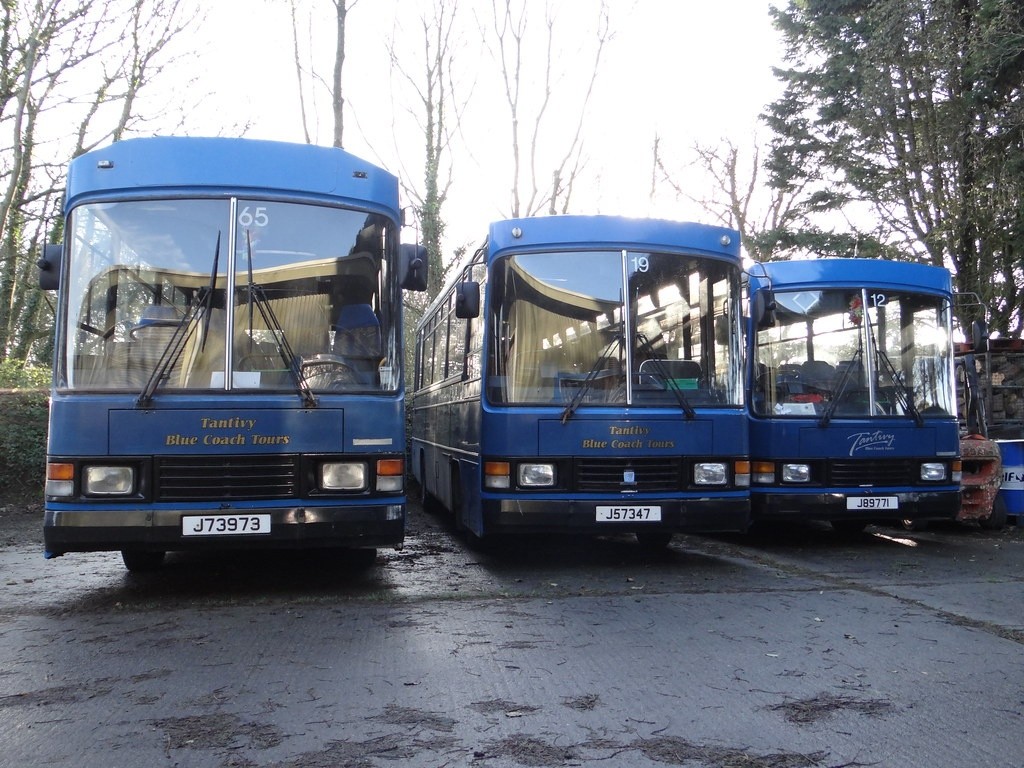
[736,261,961,528]
[36,134,430,579]
[408,213,755,561]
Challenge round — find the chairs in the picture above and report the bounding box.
[717,356,945,396]
[331,303,382,384]
[540,328,669,388]
[126,305,280,372]
